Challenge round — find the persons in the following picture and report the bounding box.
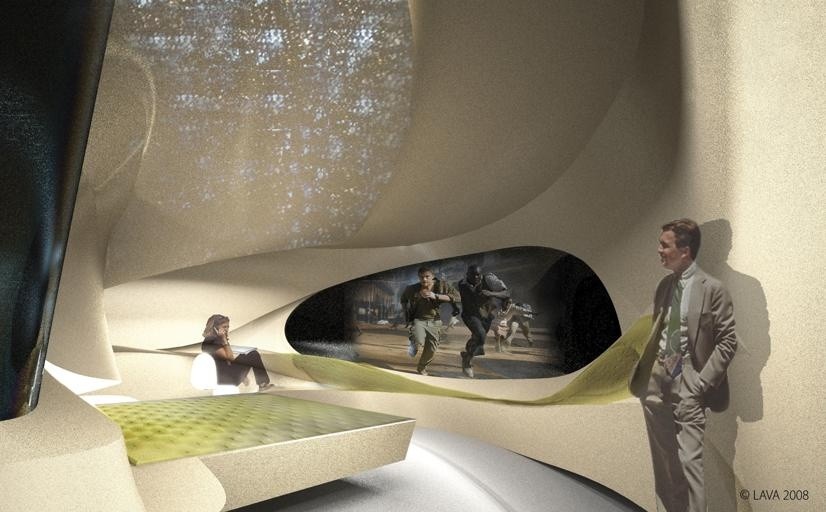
[629,219,738,512]
[400,265,536,378]
[201,314,275,392]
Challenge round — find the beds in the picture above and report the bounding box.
[94,391,418,511]
